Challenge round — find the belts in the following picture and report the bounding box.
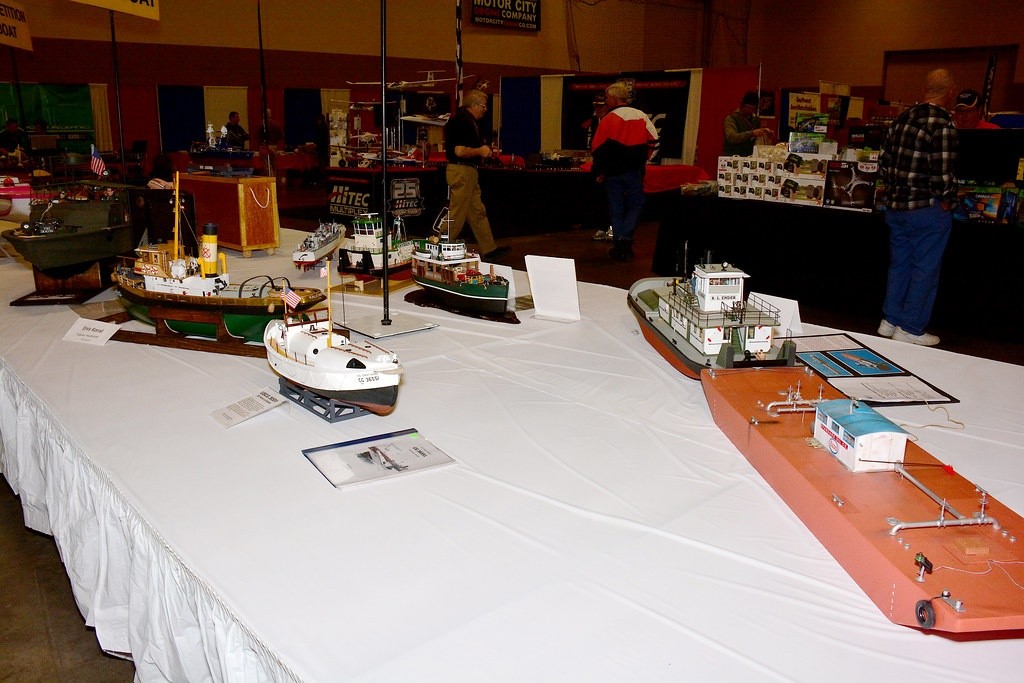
[449,161,479,169]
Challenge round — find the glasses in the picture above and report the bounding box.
[479,103,487,108]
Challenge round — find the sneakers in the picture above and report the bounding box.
[878,319,896,337]
[483,245,511,262]
[892,326,940,346]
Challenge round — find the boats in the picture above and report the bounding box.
[536,153,586,170]
[627,240,797,381]
[410,236,509,312]
[186,122,255,169]
[111,171,327,343]
[263,261,403,416]
[1,182,150,266]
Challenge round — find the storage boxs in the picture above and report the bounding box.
[716,114,1024,224]
[174,171,280,259]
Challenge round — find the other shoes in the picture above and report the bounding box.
[605,229,614,241]
[608,241,626,261]
[625,238,634,256]
[593,229,606,241]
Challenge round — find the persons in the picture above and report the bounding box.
[875,66,957,344]
[438,92,513,260]
[952,88,1001,130]
[586,91,614,242]
[256,108,281,145]
[25,119,61,156]
[314,114,330,161]
[591,81,660,260]
[723,91,775,156]
[0,118,31,156]
[225,112,250,150]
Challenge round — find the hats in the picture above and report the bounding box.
[953,89,981,111]
[921,69,955,103]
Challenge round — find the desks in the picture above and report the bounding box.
[0,229,1024,683]
[652,188,1024,346]
[327,165,711,243]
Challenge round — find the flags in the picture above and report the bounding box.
[280,287,301,308]
[91,145,105,175]
[319,268,328,278]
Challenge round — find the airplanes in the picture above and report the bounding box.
[331,99,397,111]
[356,149,423,167]
[400,113,452,126]
[346,69,474,91]
[350,131,382,142]
